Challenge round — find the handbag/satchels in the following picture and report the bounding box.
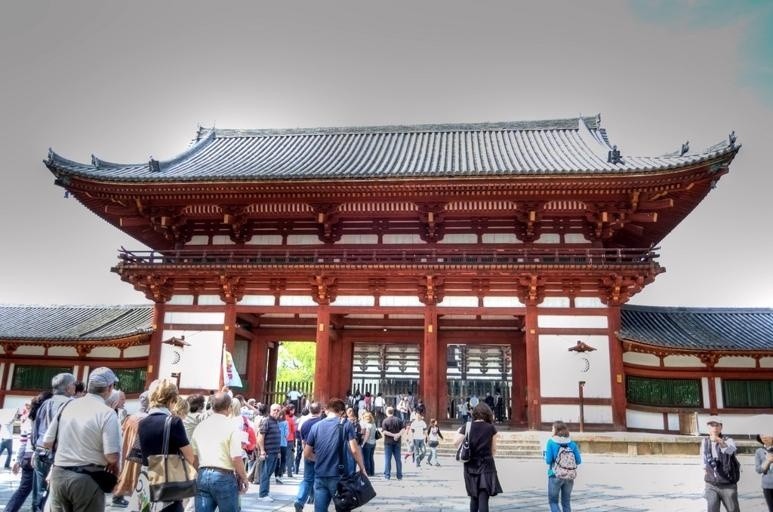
[90,470,117,492]
[333,470,376,512]
[375,430,382,440]
[148,478,197,512]
[246,456,260,484]
[456,421,471,463]
[429,441,439,447]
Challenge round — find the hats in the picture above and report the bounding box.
[51,373,77,390]
[88,367,119,388]
[705,416,724,426]
[125,429,144,464]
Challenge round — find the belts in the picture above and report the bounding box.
[202,466,224,473]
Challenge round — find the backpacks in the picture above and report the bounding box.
[552,446,577,480]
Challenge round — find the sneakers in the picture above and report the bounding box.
[111,495,129,508]
[258,496,274,503]
[426,463,433,467]
[294,502,302,512]
[275,476,283,484]
[435,463,440,466]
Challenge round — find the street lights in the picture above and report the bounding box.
[565,341,599,433]
[160,334,192,398]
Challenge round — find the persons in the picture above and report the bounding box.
[544,420,583,511]
[700,415,741,512]
[756,434,772,512]
